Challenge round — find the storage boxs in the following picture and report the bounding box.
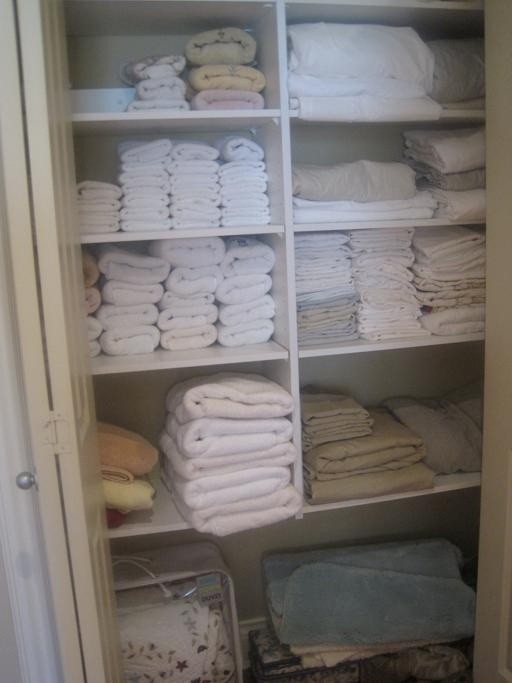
[247,627,472,682]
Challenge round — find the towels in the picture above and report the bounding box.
[116,27,268,117]
[296,230,494,355]
[265,540,477,671]
[93,374,304,536]
[77,135,272,234]
[303,389,487,509]
[78,235,279,359]
[288,122,484,218]
[286,25,485,122]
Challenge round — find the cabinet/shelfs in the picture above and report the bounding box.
[54,3,488,683]
